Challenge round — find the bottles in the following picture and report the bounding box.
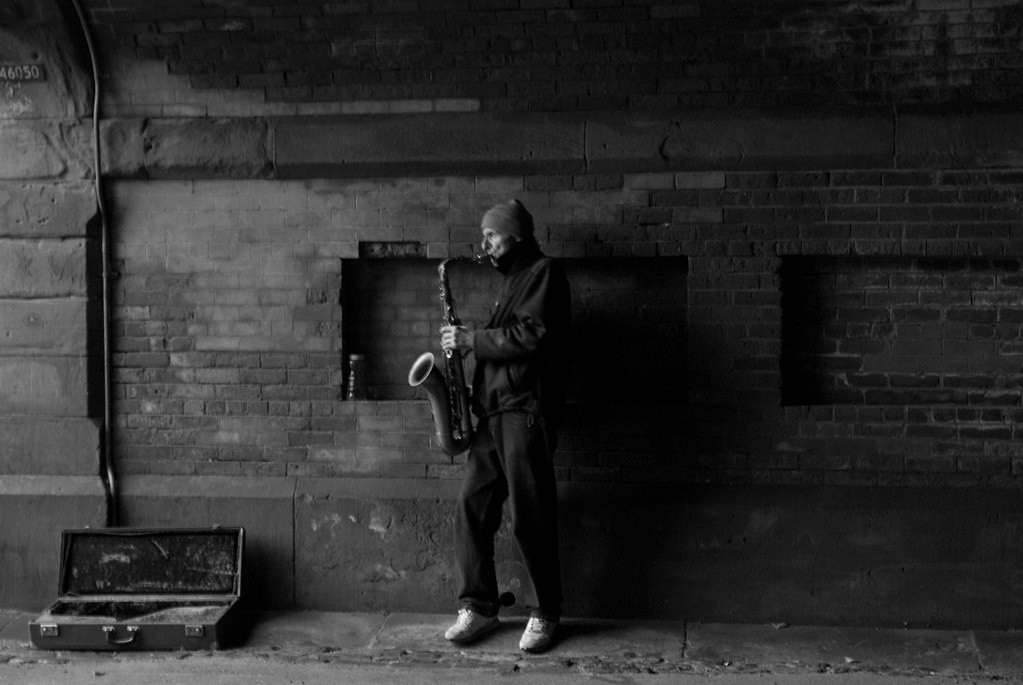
[347,354,364,400]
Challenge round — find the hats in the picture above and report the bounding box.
[481,199,535,241]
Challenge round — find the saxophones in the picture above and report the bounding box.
[406,252,495,456]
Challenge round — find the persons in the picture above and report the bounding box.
[439,197,580,650]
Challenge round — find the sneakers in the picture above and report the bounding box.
[519,615,558,652]
[445,608,498,642]
